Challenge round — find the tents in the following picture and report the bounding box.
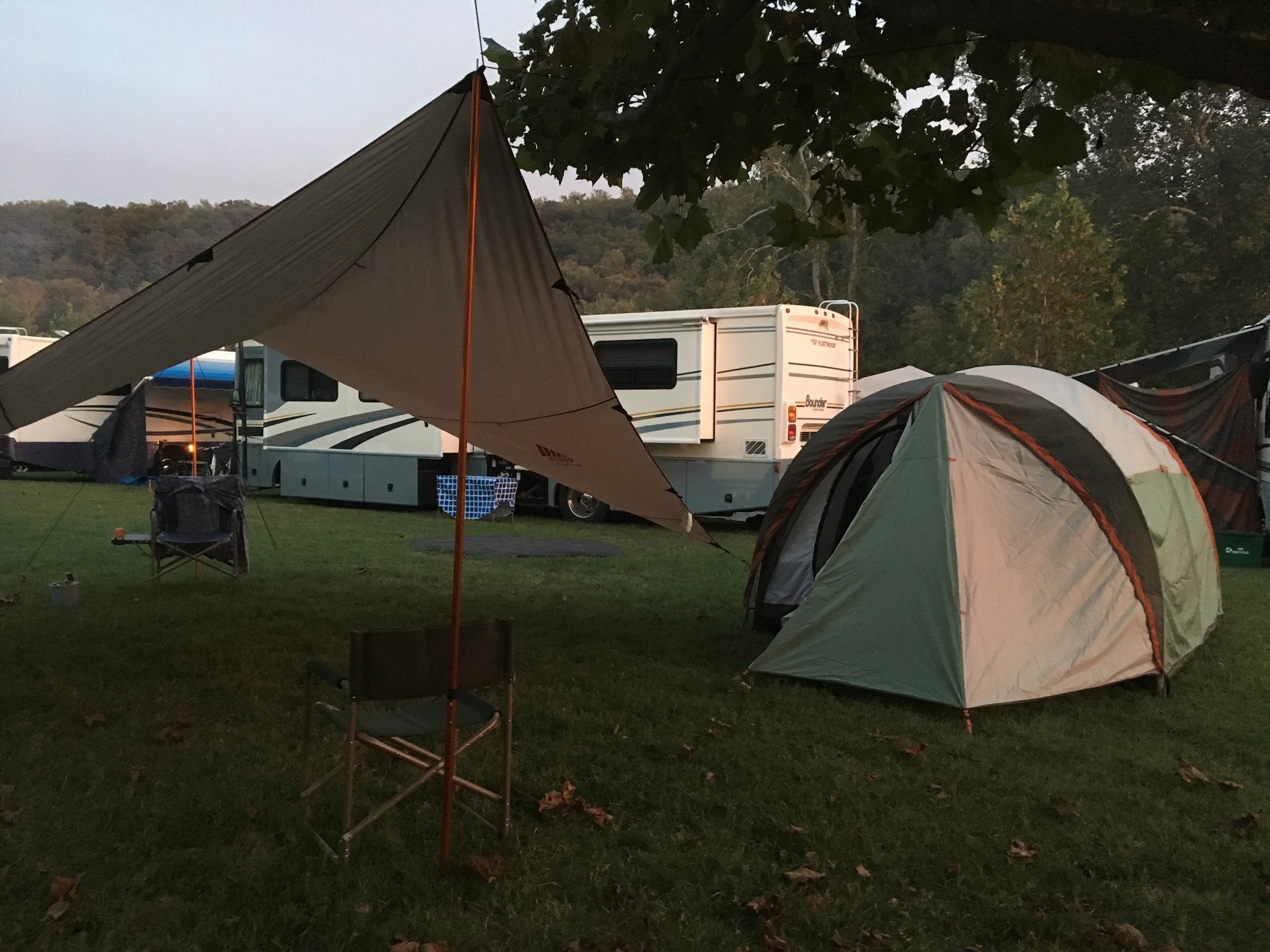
[744,364,1226,730]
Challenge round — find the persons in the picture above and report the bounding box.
[153,445,164,476]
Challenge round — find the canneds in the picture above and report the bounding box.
[114,528,124,540]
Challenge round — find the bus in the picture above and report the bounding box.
[0,325,236,481]
[229,296,859,524]
